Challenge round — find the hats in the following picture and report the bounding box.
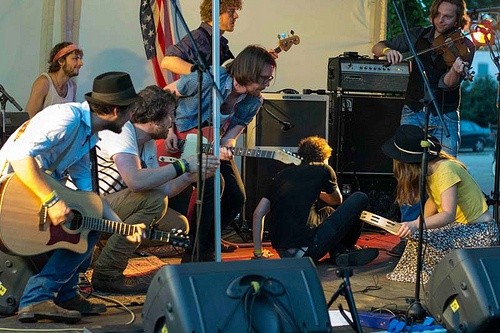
[85,72,142,105]
[382,124,442,163]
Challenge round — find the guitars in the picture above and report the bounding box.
[154,127,304,168]
[0,172,191,257]
[221,30,300,68]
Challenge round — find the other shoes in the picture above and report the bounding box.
[221,243,238,250]
[335,248,380,266]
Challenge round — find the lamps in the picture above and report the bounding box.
[471,10,500,47]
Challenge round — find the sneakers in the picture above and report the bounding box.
[18,300,81,322]
[53,294,107,313]
[91,276,147,294]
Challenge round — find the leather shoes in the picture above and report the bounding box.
[296,246,309,258]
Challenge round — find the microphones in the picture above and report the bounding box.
[470,24,489,34]
[281,125,291,133]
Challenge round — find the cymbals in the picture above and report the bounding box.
[360,211,401,236]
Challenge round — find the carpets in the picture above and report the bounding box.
[79,239,168,305]
[221,234,401,266]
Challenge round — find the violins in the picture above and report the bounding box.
[431,30,475,82]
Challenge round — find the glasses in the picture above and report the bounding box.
[225,7,236,16]
[259,76,273,84]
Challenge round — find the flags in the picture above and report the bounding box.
[139,0,181,89]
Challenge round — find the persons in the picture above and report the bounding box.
[251,136,380,268]
[24,41,83,121]
[372,0,476,258]
[160,0,278,252]
[91,85,220,295]
[161,45,277,263]
[381,124,500,286]
[0,71,149,324]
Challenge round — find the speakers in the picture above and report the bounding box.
[142,258,334,333]
[0,247,54,314]
[245,90,330,152]
[331,92,405,174]
[423,244,500,333]
[241,153,328,223]
[336,174,400,233]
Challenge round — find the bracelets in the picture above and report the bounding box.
[41,190,61,208]
[252,249,263,255]
[173,158,190,178]
[383,48,391,56]
